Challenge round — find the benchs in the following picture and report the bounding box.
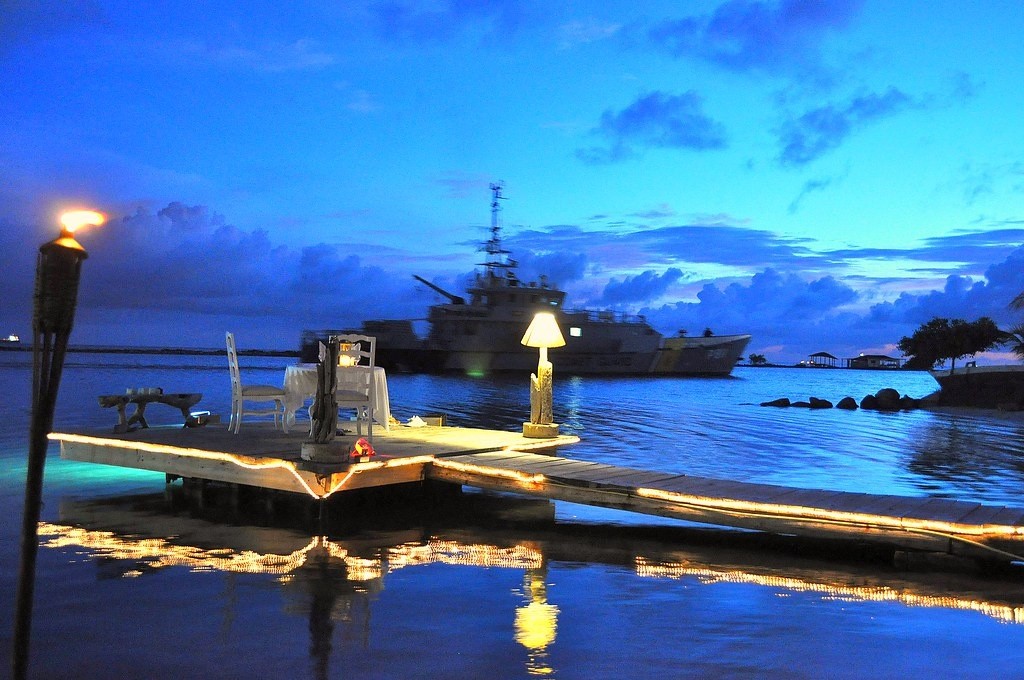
[97,390,204,434]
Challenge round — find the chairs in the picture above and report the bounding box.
[328,333,378,446]
[224,330,289,437]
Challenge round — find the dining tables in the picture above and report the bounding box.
[282,361,391,445]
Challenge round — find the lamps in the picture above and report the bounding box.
[520,310,566,439]
[340,343,356,366]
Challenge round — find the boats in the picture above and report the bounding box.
[928,364,1024,398]
[297,181,751,380]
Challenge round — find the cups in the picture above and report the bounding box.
[126,388,161,395]
